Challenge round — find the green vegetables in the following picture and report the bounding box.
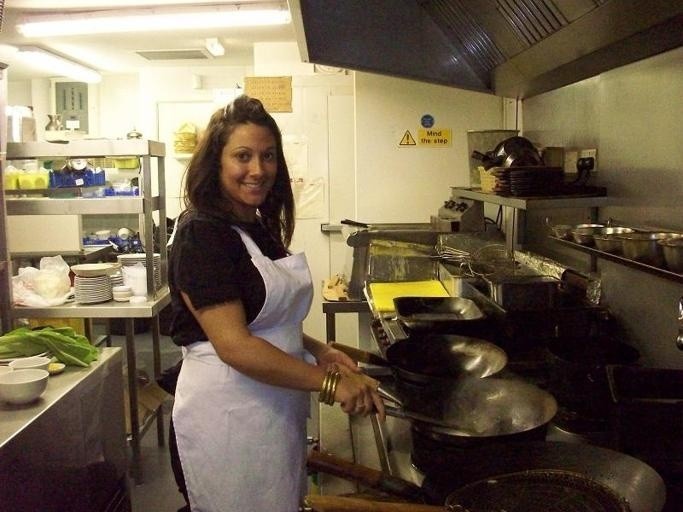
[0,325,101,368]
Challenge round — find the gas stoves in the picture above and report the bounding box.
[369,331,682,512]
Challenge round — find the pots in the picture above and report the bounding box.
[321,332,510,380]
[305,442,668,512]
[472,136,541,168]
[372,377,558,453]
[551,219,683,272]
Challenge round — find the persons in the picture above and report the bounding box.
[163,94,386,512]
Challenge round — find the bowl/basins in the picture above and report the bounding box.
[0,356,50,408]
[117,227,129,239]
[95,229,110,240]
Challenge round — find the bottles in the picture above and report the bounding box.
[43,113,64,143]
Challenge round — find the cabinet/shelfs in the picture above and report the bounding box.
[0,140,172,485]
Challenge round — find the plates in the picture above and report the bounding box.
[492,167,564,196]
[48,361,65,375]
[70,252,161,304]
[393,295,484,330]
[0,350,50,367]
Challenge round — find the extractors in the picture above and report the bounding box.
[283,0,683,101]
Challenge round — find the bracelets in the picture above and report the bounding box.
[316,368,342,406]
